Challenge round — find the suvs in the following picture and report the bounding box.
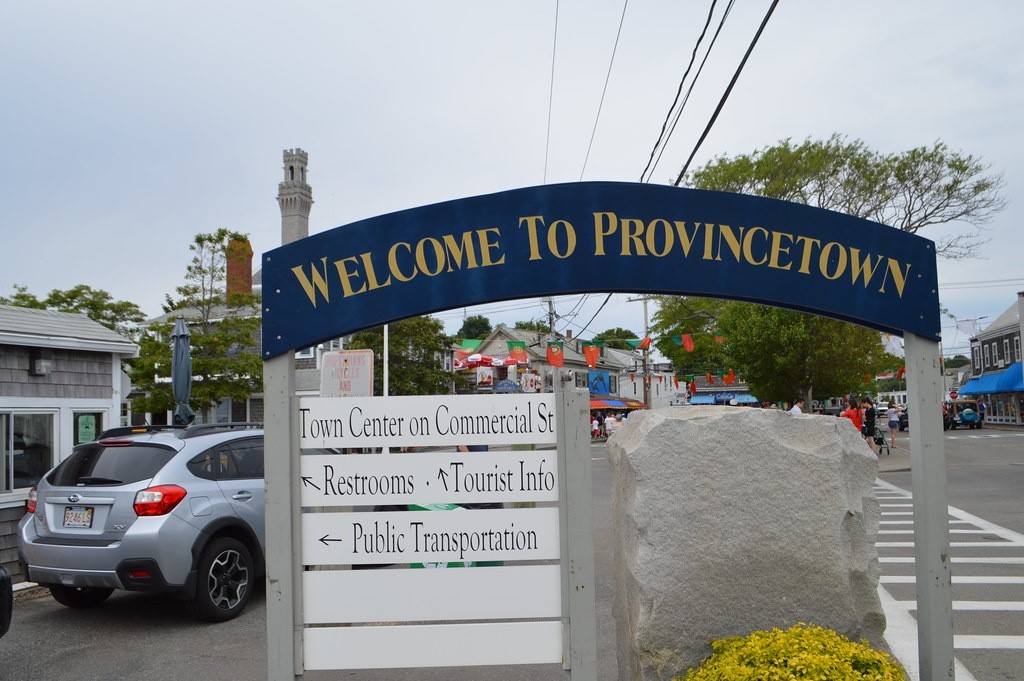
[949,399,982,429]
[16,423,266,624]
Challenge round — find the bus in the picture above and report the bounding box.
[877,402,890,418]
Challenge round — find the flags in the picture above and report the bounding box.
[862,363,905,383]
[629,369,736,393]
[625,330,726,352]
[454,339,605,371]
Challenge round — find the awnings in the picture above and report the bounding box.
[690,394,758,402]
[589,398,647,409]
[957,361,1024,395]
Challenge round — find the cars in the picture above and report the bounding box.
[899,405,950,432]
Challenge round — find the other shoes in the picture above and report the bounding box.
[891,444,898,448]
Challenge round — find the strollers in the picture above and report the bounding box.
[874,427,890,455]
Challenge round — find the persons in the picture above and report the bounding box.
[839,395,877,452]
[478,372,492,387]
[524,375,533,389]
[750,402,778,409]
[978,398,987,425]
[887,400,900,448]
[790,399,804,416]
[988,398,1009,416]
[590,412,627,439]
[457,445,488,452]
[943,400,952,431]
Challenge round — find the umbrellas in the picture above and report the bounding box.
[454,360,462,374]
[505,357,529,365]
[170,314,195,426]
[456,353,504,371]
